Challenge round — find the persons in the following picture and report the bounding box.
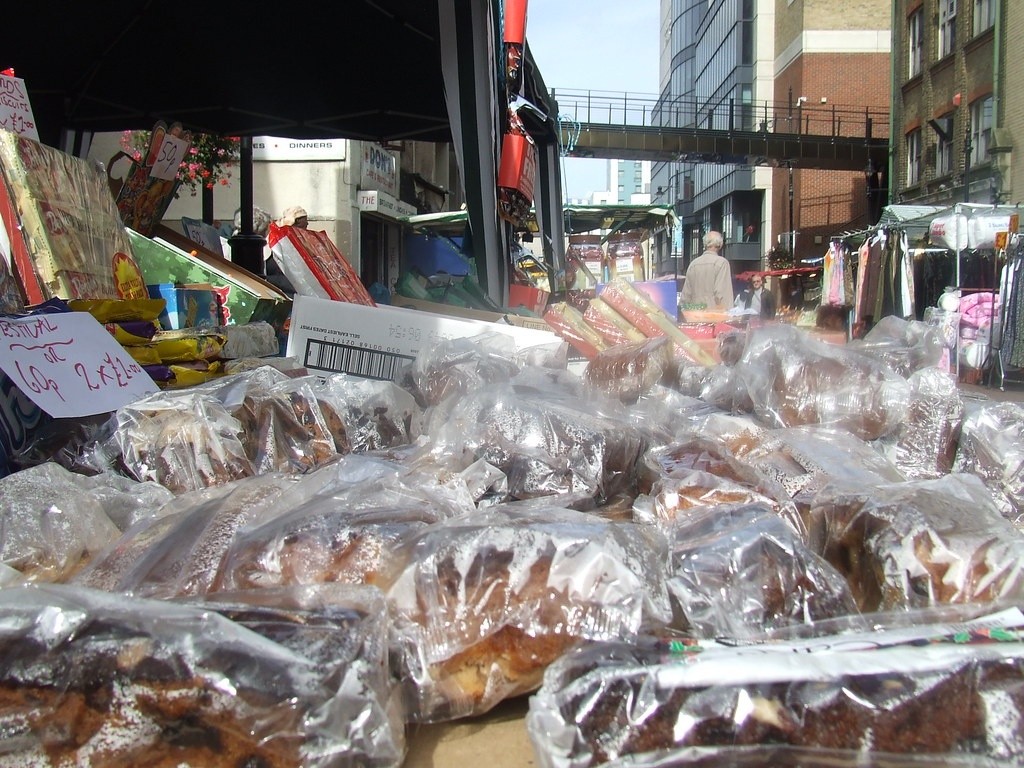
[745,274,776,319]
[734,285,752,310]
[276,206,308,230]
[679,231,733,310]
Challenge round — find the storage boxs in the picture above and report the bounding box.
[144,283,219,330]
[124,223,291,330]
[288,294,568,395]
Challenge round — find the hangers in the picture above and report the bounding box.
[832,237,848,245]
[860,228,906,247]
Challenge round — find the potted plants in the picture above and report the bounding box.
[765,246,805,310]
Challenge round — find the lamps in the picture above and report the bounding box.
[655,186,677,195]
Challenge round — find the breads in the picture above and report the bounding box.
[0,321,1024,768]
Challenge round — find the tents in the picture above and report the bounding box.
[1,0,567,310]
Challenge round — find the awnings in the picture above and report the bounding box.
[399,203,678,271]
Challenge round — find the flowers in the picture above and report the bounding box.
[120,131,240,200]
